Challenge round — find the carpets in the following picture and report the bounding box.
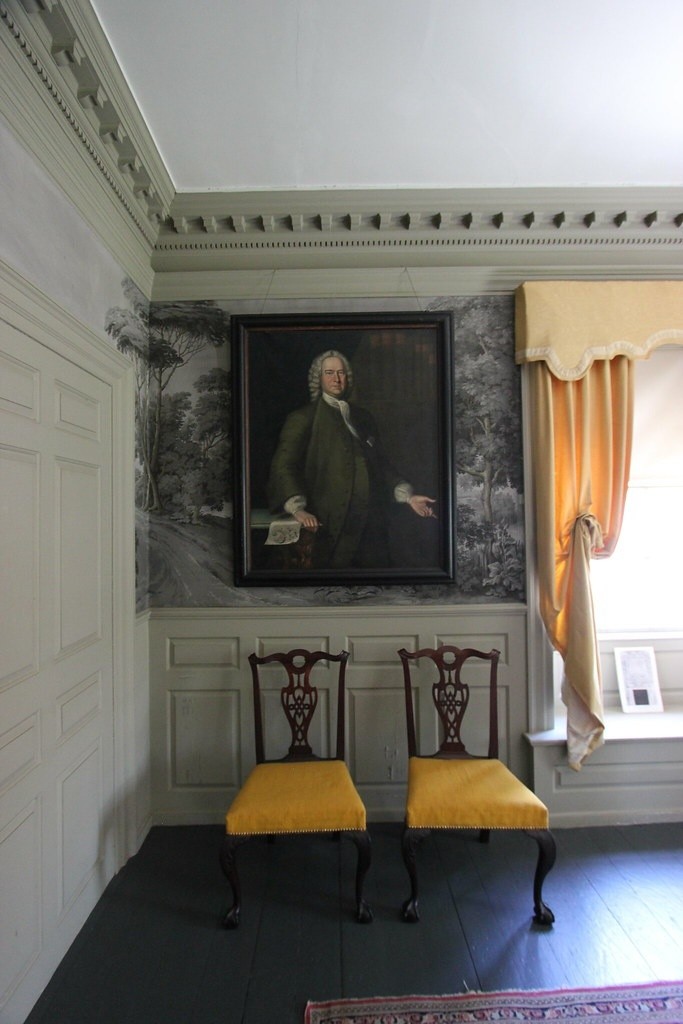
[303,975,683,1024]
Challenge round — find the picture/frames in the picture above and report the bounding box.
[614,646,664,714]
[231,310,456,587]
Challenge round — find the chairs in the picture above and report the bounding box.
[397,645,556,933]
[225,648,372,930]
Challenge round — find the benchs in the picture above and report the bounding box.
[523,711,682,828]
